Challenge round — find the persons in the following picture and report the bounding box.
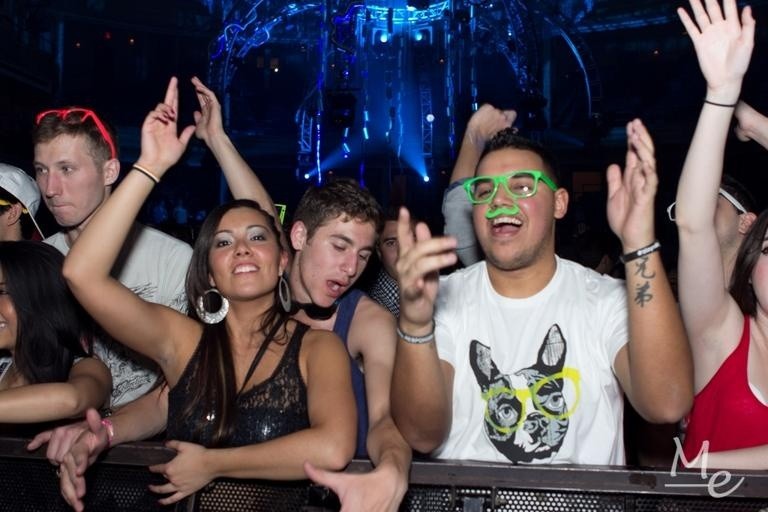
[0,240,112,442]
[28,106,196,463]
[63,75,357,511]
[364,208,421,315]
[674,1,768,468]
[61,175,412,511]
[667,161,760,299]
[390,115,694,512]
[442,103,516,264]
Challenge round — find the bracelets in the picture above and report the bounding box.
[617,240,660,263]
[131,162,160,185]
[702,99,738,108]
[394,320,435,343]
[99,418,113,445]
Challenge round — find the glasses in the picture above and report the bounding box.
[480,366,581,433]
[717,188,747,214]
[667,200,679,220]
[36,108,116,159]
[280,300,338,320]
[463,170,556,204]
